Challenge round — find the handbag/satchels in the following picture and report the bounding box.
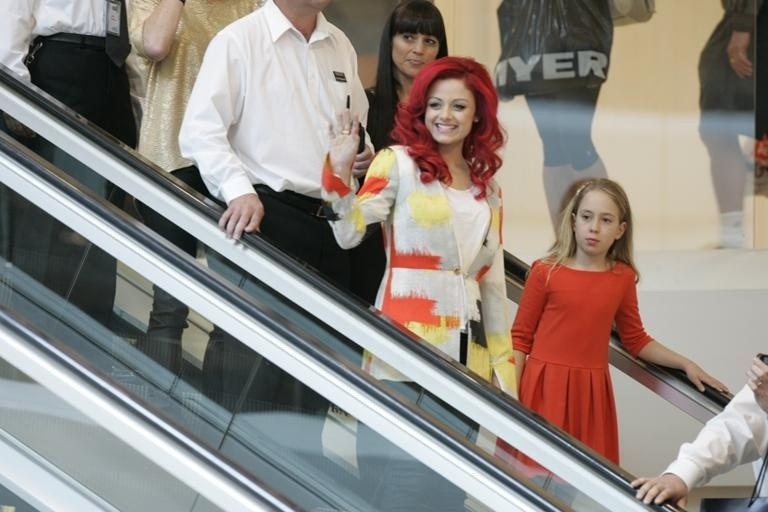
[607,0,655,26]
[700,497,768,512]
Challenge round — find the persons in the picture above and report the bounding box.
[359,0,449,191]
[124,0,268,405]
[322,56,519,511]
[492,178,729,507]
[0,0,137,327]
[494,0,612,242]
[630,353,768,512]
[698,0,757,248]
[179,0,376,470]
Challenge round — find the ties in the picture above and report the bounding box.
[106,0,130,68]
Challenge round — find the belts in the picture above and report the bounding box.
[44,33,105,47]
[253,184,327,218]
